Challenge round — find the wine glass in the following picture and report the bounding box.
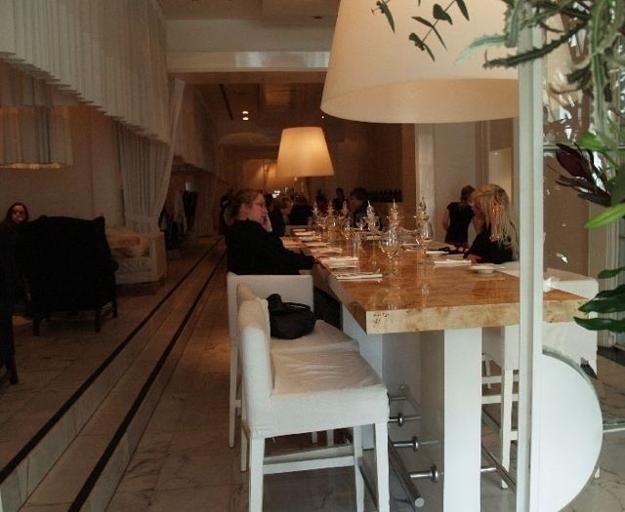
[355,213,367,231]
[379,229,400,278]
[419,222,435,258]
[341,218,354,244]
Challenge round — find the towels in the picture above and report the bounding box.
[423,249,451,254]
[292,228,343,254]
[332,271,383,280]
[431,258,473,264]
[320,255,359,262]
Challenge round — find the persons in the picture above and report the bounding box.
[0,203,31,294]
[441,185,479,249]
[215,189,343,330]
[348,186,384,233]
[464,185,519,263]
[332,186,345,210]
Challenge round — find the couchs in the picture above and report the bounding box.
[106,223,165,296]
[42,215,121,332]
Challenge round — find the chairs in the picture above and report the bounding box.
[481,267,600,488]
[239,300,388,512]
[234,284,360,471]
[226,270,316,448]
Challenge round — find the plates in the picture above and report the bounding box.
[471,265,503,274]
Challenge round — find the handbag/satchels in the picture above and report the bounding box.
[266,292,318,339]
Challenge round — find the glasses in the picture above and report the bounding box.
[246,201,266,209]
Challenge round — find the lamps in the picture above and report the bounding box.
[275,126,334,195]
[320,0,581,125]
